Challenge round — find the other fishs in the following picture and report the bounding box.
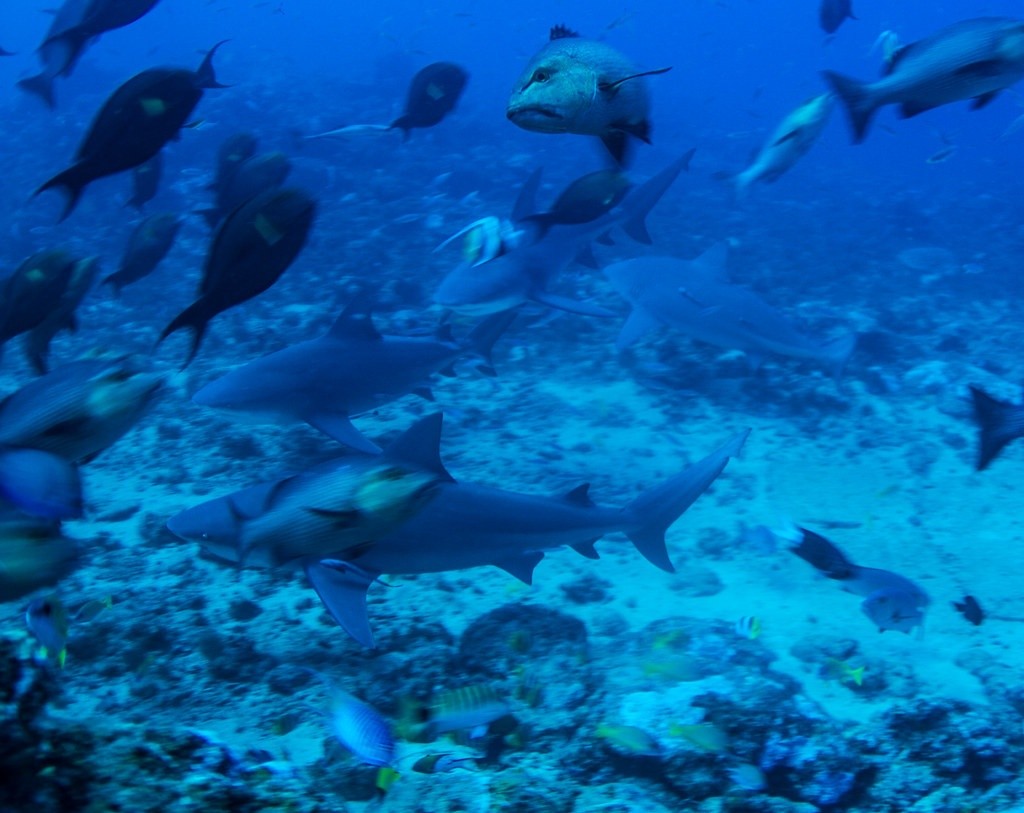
[0,2,1024,803]
[510,23,673,163]
[150,188,321,370]
